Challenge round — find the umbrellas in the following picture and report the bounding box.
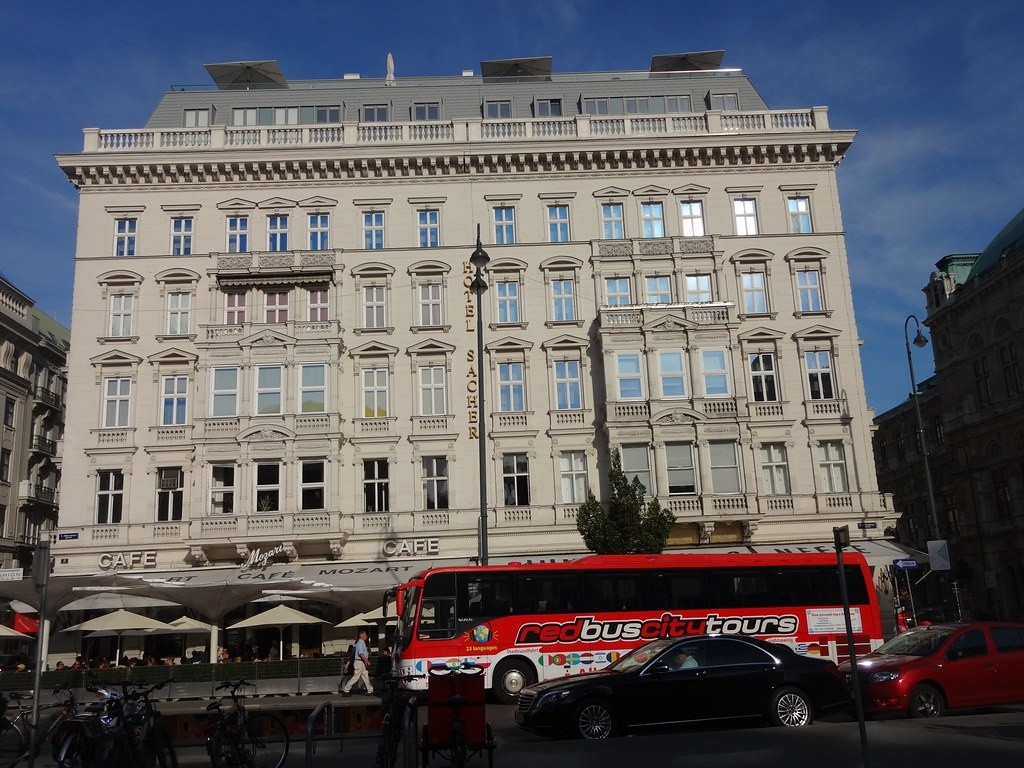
[0,623,36,640]
[59,609,222,666]
[226,604,333,660]
[334,599,436,652]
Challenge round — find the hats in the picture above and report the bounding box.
[17,664,25,669]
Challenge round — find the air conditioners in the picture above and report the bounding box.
[160,478,178,489]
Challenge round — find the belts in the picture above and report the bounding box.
[355,659,363,661]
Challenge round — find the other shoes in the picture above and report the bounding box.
[368,692,375,695]
[341,691,351,697]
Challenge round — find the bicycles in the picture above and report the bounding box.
[0,679,178,768]
[369,672,426,768]
[205,680,289,768]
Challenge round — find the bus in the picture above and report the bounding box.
[382,552,884,706]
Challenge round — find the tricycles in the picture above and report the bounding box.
[419,663,498,768]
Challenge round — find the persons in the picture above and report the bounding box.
[383,647,392,695]
[56,656,177,670]
[182,637,325,664]
[668,649,699,670]
[0,652,35,672]
[342,631,379,696]
[897,604,909,633]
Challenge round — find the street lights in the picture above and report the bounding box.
[469,222,491,565]
[904,314,941,541]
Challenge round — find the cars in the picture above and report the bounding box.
[514,634,852,741]
[836,621,1024,719]
